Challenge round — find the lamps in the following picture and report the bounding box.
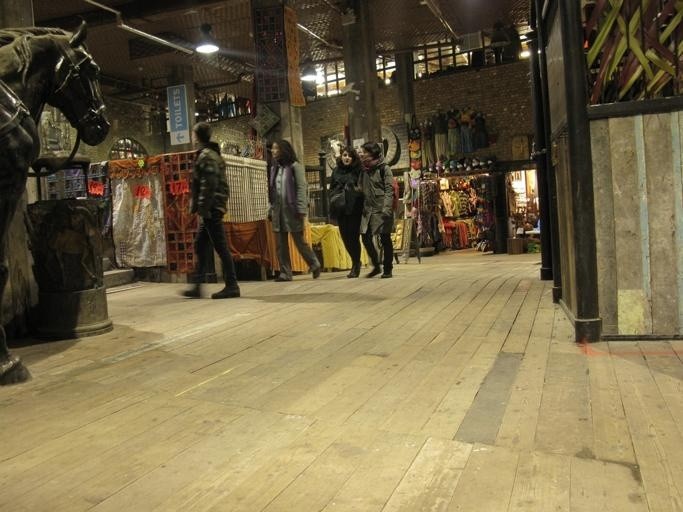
[300,57,317,82]
[195,23,220,54]
[340,8,356,26]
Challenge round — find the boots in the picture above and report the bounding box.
[346,261,361,278]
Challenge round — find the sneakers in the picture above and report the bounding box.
[275,276,285,281]
[313,268,320,279]
[212,286,240,298]
[368,268,381,278]
[182,289,200,297]
[382,273,392,278]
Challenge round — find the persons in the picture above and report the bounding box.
[328,147,368,278]
[181,121,242,298]
[266,138,322,281]
[359,140,395,278]
[419,107,488,171]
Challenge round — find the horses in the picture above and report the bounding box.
[0,19,112,385]
